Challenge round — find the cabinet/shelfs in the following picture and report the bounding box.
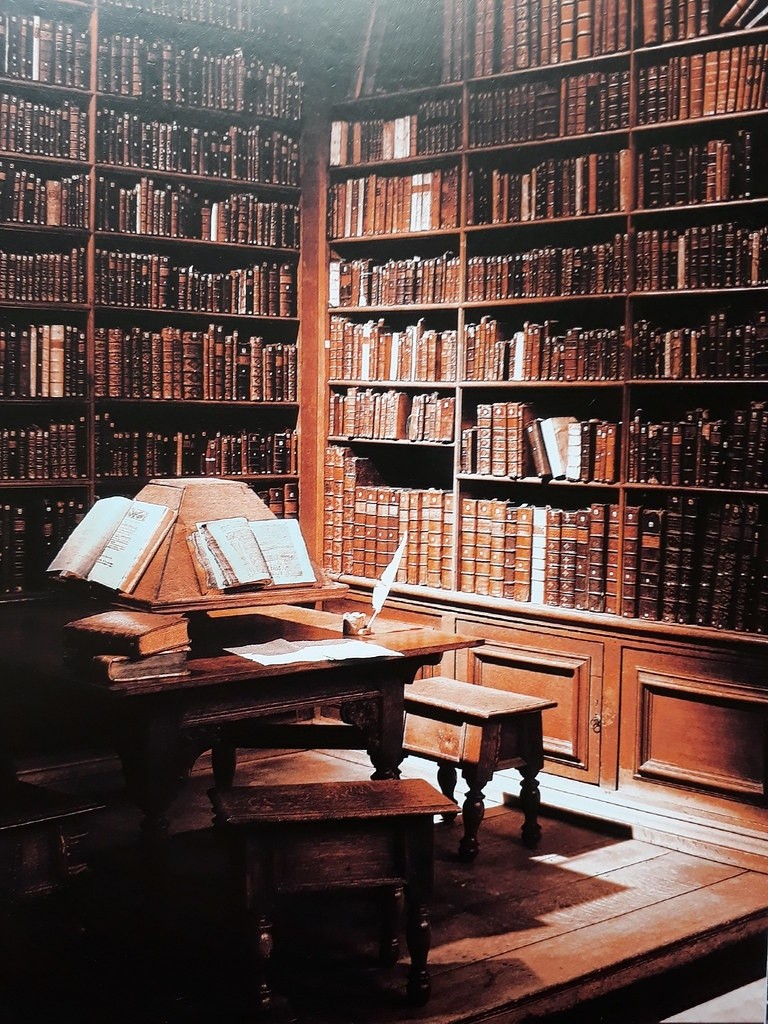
[0,1,768,880]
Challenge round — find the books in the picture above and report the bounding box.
[346,0,768,98]
[0,324,298,401]
[223,638,405,666]
[325,314,768,381]
[0,0,307,115]
[0,163,300,251]
[63,611,192,681]
[0,93,299,186]
[0,244,295,318]
[324,445,452,590]
[328,222,768,307]
[0,413,297,479]
[329,387,456,441]
[0,483,317,597]
[330,44,768,164]
[461,401,768,490]
[321,130,752,239]
[460,496,768,635]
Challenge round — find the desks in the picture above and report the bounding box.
[55,607,486,819]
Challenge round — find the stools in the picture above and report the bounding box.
[398,676,558,868]
[168,781,462,1024]
